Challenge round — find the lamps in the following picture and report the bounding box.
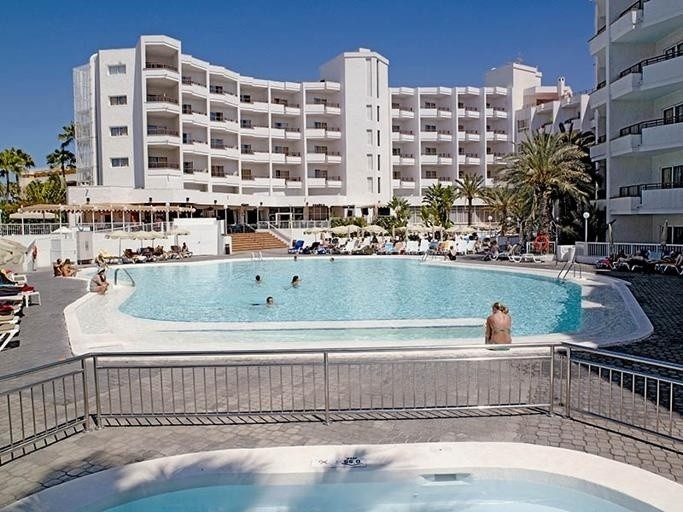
[104,246,193,264]
[593,251,683,276]
[0,274,41,352]
[288,236,554,264]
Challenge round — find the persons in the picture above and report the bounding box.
[294,251,299,261]
[90,266,109,294]
[292,276,298,283]
[599,249,683,275]
[320,230,514,262]
[32,245,36,271]
[485,302,511,350]
[256,275,260,281]
[267,296,273,304]
[0,271,33,331]
[95,253,104,267]
[54,259,62,276]
[62,259,81,276]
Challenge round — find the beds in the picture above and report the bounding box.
[329,225,389,238]
[105,229,191,256]
[0,238,28,267]
[661,219,668,245]
[395,224,476,239]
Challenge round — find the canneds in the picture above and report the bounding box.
[534,236,549,252]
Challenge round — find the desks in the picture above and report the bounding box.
[288,200,292,221]
[517,217,520,228]
[16,198,195,236]
[224,201,227,236]
[254,202,261,229]
[506,216,511,235]
[582,212,590,242]
[487,215,492,237]
[327,203,331,222]
